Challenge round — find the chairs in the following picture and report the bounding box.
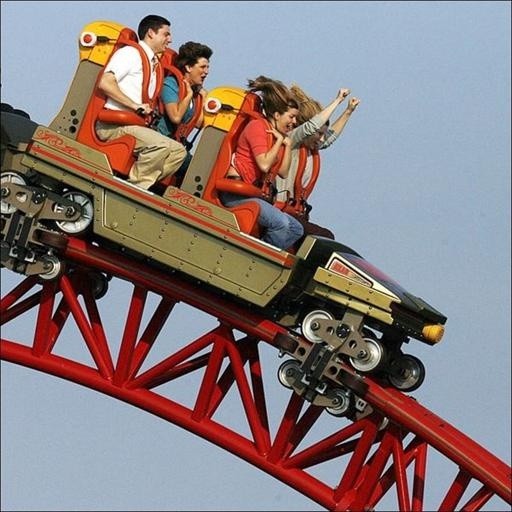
[75,28,164,175]
[277,133,321,216]
[202,94,285,235]
[160,47,202,185]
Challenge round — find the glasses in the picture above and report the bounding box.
[285,100,298,109]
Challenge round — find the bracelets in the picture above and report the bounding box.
[334,94,345,103]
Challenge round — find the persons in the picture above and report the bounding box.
[150,40,214,189]
[218,73,304,251]
[275,81,360,250]
[94,14,188,191]
